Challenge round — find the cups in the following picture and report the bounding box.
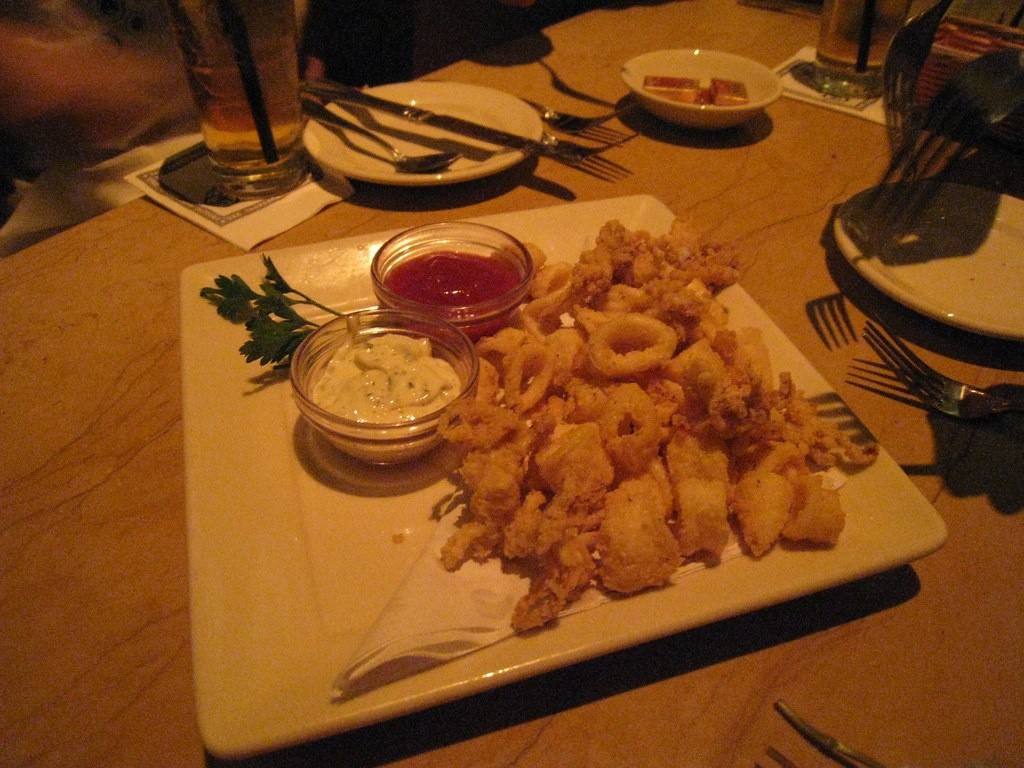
[168,0,304,202]
[813,0,917,98]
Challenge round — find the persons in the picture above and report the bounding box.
[0,0,336,255]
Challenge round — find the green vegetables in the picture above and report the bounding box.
[198,251,349,369]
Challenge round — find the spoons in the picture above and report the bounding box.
[302,98,464,174]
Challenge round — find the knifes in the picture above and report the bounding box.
[298,78,582,165]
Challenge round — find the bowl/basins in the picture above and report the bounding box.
[290,311,479,464]
[620,50,782,131]
[371,222,533,340]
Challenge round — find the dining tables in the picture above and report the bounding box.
[0,0,1024,768]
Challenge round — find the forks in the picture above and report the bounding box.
[867,49,1024,236]
[520,98,634,134]
[545,134,641,165]
[882,0,950,155]
[864,313,1024,419]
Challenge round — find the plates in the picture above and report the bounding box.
[302,82,543,186]
[834,178,1024,340]
[177,194,947,756]
